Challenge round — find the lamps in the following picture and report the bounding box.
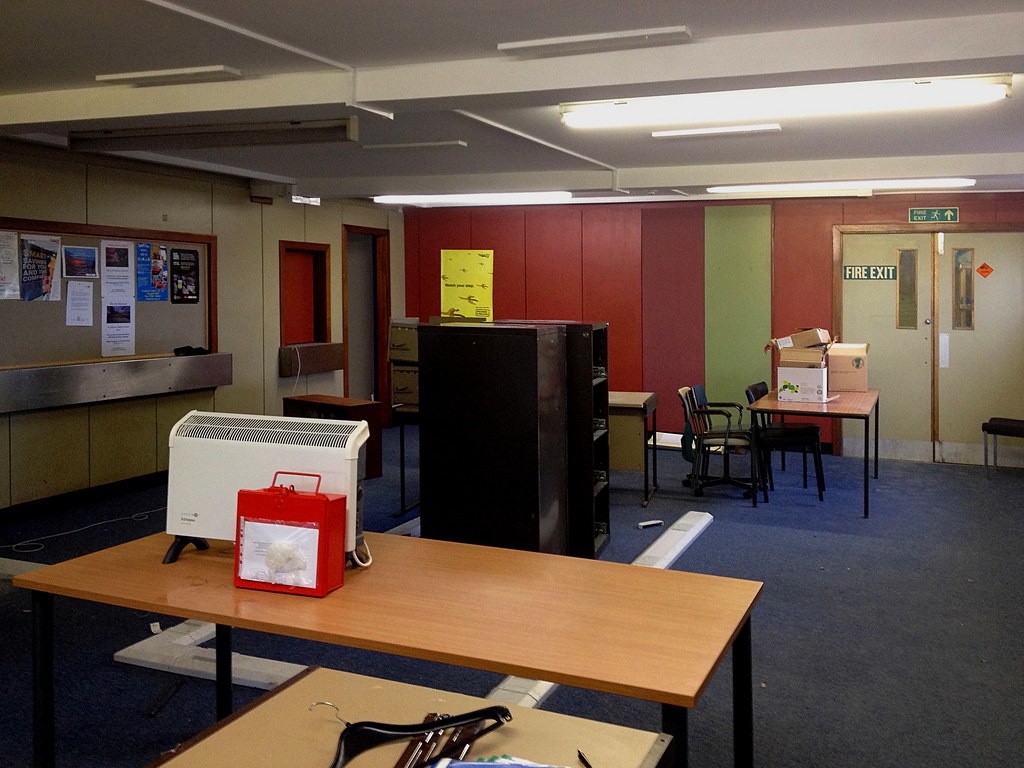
[559,73,1014,130]
[67,115,359,156]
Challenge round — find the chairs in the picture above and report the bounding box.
[746,381,826,503]
[676,387,764,500]
[981,417,1024,482]
[693,384,770,488]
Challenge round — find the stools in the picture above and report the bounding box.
[282,394,384,481]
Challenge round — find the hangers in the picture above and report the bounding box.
[309,701,514,768]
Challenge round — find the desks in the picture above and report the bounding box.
[12,530,767,768]
[608,391,660,507]
[746,386,878,519]
[145,665,675,768]
[393,403,418,519]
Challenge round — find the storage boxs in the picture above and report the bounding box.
[387,315,487,364]
[390,362,418,414]
[778,335,839,369]
[777,367,828,403]
[764,327,832,352]
[826,343,871,392]
